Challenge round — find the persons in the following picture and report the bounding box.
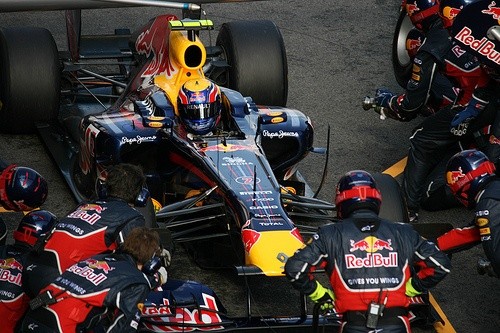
[169,81,296,208]
[0,158,227,333]
[428,150,500,279]
[373,0,500,223]
[284,170,452,333]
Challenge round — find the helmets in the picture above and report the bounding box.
[335,170,383,218]
[405,28,426,60]
[176,78,222,136]
[0,163,48,212]
[444,149,498,209]
[437,0,467,30]
[402,0,441,29]
[13,209,62,251]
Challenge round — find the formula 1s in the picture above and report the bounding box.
[0,1,349,333]
